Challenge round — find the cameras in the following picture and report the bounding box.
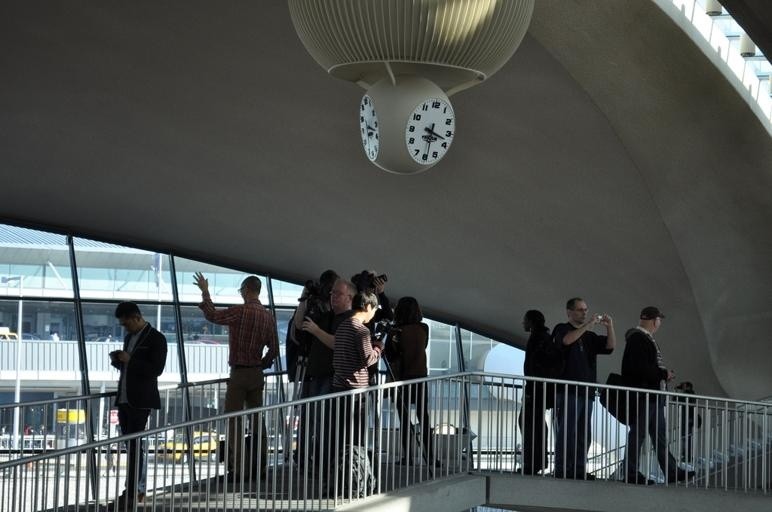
[668,369,675,379]
[305,279,331,302]
[677,384,683,389]
[375,319,402,338]
[368,274,387,288]
[111,352,121,359]
[595,314,604,321]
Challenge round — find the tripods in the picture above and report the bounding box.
[283,302,318,462]
[369,338,433,494]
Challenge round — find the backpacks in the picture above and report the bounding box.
[331,443,378,500]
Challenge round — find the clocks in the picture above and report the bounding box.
[359,95,380,162]
[403,97,455,166]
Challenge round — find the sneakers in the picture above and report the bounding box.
[623,470,697,485]
[112,487,145,508]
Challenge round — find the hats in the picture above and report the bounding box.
[639,306,666,320]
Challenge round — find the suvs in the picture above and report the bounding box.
[157,433,216,463]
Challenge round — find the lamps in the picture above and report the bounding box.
[704,0,722,17]
[738,33,754,58]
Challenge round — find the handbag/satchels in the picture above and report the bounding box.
[693,413,701,428]
[597,371,632,428]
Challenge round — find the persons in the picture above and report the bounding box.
[383,296,443,468]
[322,291,388,497]
[517,309,568,474]
[286,269,339,479]
[621,307,696,485]
[1,425,7,434]
[294,279,358,385]
[39,424,45,435]
[671,381,702,462]
[552,297,617,480]
[108,302,168,503]
[192,272,280,483]
